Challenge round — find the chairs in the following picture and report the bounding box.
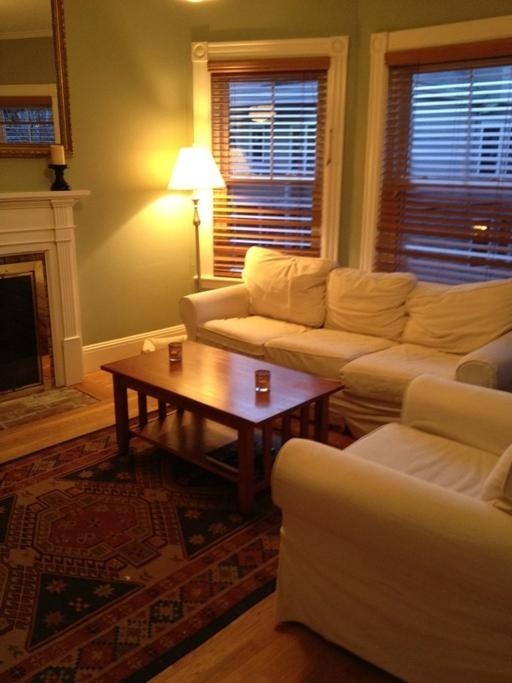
[268,372,512,682]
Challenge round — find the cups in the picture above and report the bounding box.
[254,368,271,393]
[168,342,183,363]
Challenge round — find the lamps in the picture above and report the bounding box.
[168,146,228,290]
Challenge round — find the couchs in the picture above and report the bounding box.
[176,245,512,440]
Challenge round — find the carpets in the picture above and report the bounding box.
[0,406,358,682]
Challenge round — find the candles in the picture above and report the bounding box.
[49,143,66,164]
